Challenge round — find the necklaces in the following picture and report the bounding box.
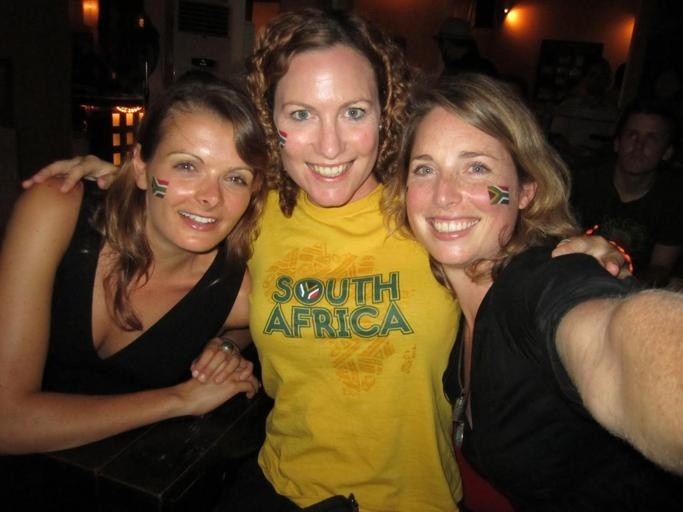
[449,314,470,422]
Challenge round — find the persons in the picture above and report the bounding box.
[20,2,636,512]
[572,98,683,280]
[0,71,273,458]
[378,66,683,512]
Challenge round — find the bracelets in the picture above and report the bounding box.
[586,223,634,274]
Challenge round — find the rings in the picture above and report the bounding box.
[221,341,234,354]
